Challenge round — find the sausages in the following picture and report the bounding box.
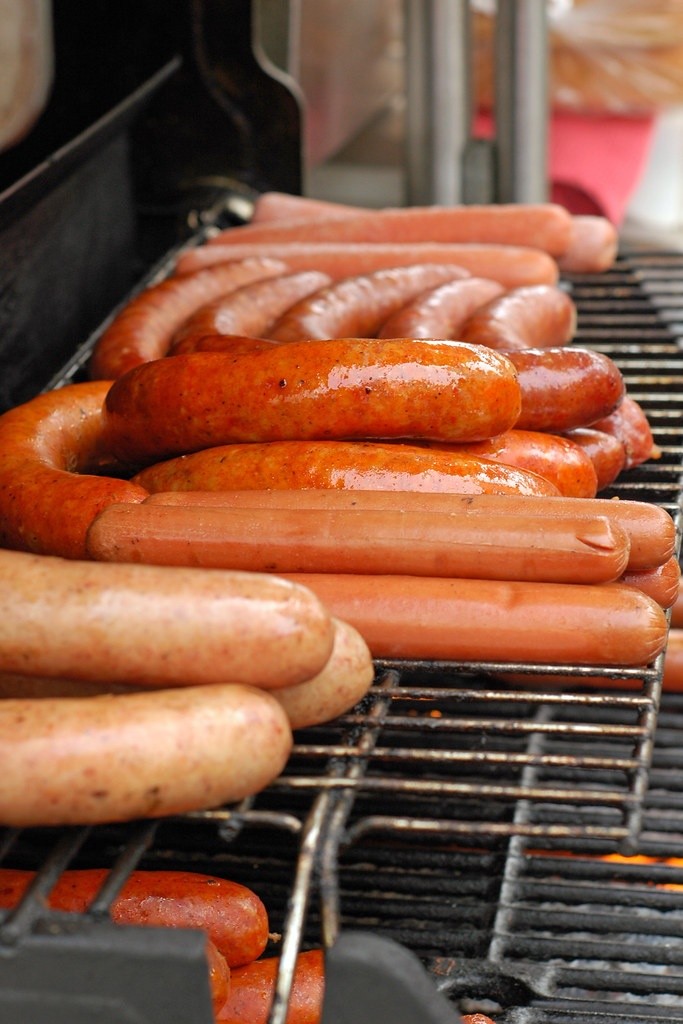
[0,868,325,1024]
[459,1013,495,1024]
[0,190,683,827]
